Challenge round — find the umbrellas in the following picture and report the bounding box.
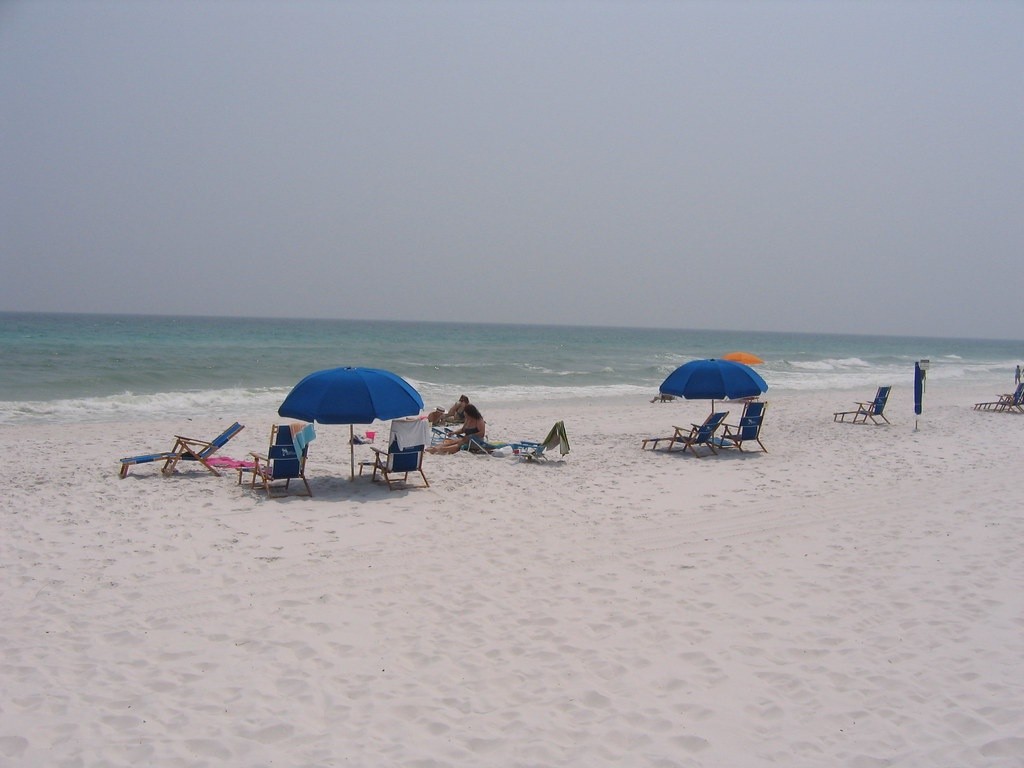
[914,362,923,429]
[721,352,764,364]
[278,367,424,479]
[659,358,768,451]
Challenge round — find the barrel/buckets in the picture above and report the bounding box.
[366,432,375,443]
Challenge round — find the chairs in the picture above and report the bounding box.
[236,424,317,498]
[429,428,512,457]
[833,386,892,425]
[973,383,1024,415]
[357,416,433,491]
[707,401,769,453]
[642,411,731,459]
[117,420,246,478]
[512,420,570,463]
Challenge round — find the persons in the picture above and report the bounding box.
[426,405,485,454]
[1015,365,1024,385]
[649,393,673,403]
[406,407,445,427]
[444,396,468,422]
